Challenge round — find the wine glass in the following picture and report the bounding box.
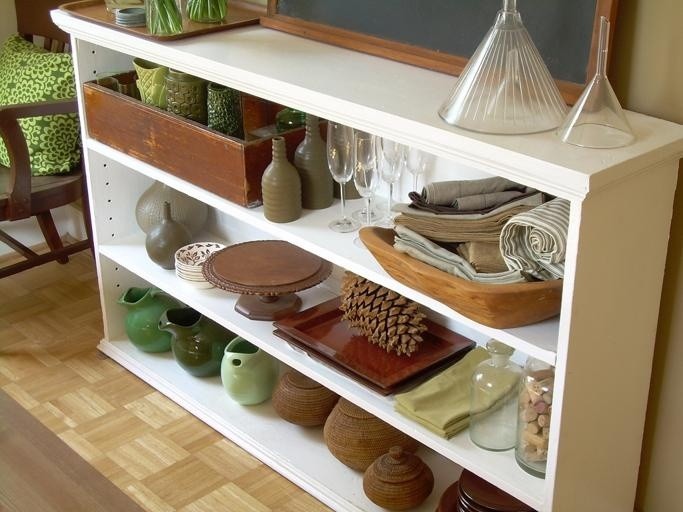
[325,118,425,235]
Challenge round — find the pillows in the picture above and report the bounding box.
[0,32,81,174]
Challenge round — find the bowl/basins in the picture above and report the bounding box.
[116,8,146,28]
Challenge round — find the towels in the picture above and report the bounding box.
[388,177,570,286]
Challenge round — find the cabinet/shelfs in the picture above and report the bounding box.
[50,0,683,512]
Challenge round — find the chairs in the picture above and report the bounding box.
[0,1,95,278]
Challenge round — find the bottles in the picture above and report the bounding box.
[470,336,517,452]
[105,1,145,13]
[147,0,229,34]
[119,287,279,408]
[516,358,554,479]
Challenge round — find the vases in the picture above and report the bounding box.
[185,0,228,24]
[162,66,205,123]
[145,0,182,35]
[130,56,167,109]
[204,82,243,141]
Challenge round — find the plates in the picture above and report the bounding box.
[435,468,534,512]
[175,242,230,289]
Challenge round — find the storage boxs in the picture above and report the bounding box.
[82,71,329,213]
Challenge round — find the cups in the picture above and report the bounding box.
[130,58,245,139]
